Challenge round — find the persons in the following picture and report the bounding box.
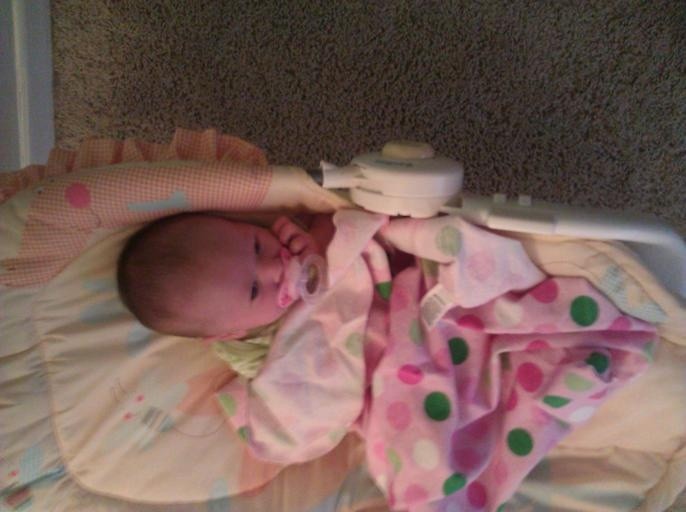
[117,209,340,385]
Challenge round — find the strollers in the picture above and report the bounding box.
[1,139,686,511]
